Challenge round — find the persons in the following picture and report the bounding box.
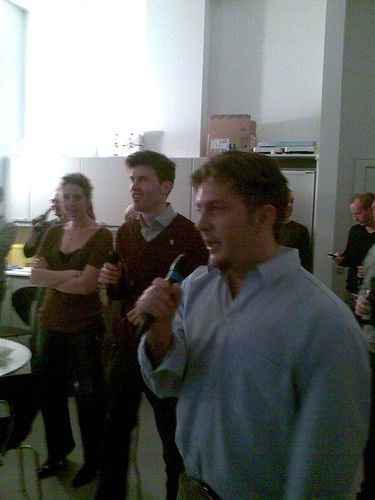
[357,197,375,500]
[12,188,72,329]
[272,188,314,274]
[0,186,17,304]
[137,150,371,500]
[96,150,210,500]
[30,173,117,489]
[327,192,375,327]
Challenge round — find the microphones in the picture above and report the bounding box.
[140,253,195,328]
[106,251,119,295]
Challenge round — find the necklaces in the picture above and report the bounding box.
[67,218,89,240]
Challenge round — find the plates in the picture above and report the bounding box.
[0,338,32,378]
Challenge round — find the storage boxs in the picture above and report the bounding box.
[206,115,256,157]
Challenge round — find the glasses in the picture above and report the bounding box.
[51,199,60,203]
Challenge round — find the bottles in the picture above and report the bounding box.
[360,286,372,323]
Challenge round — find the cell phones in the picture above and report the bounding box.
[328,253,337,257]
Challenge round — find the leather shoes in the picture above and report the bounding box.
[72,463,98,488]
[38,458,69,479]
